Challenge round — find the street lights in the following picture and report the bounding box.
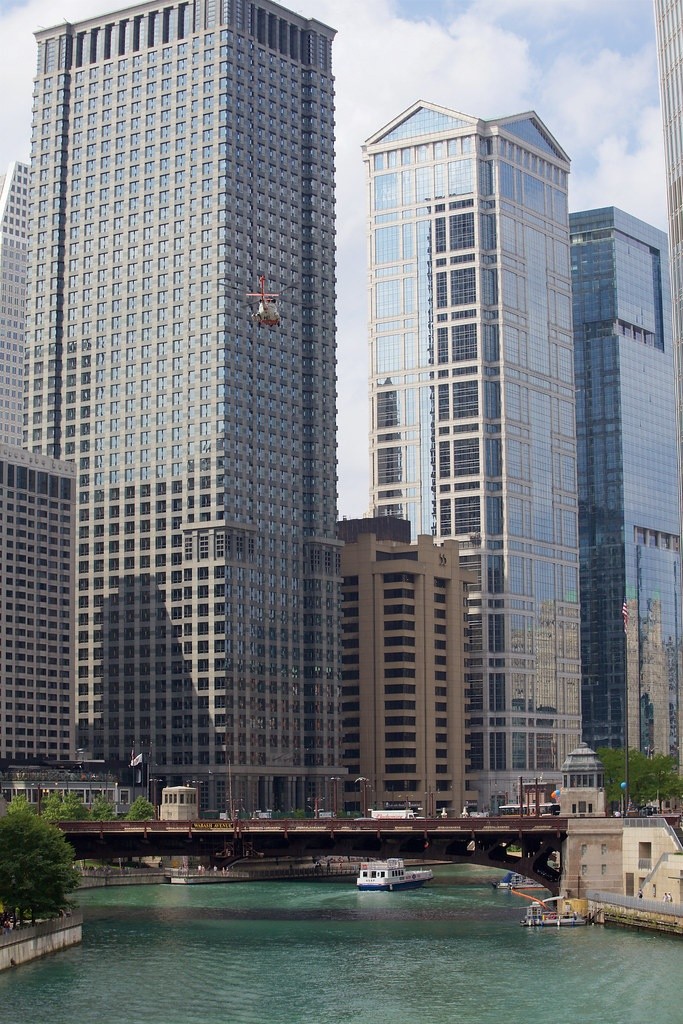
[149,778,163,820]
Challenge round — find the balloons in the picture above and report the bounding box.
[621,782,626,789]
[551,790,560,798]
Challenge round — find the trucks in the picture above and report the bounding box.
[370,810,417,819]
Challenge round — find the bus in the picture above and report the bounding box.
[498,802,559,816]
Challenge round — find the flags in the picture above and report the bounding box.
[130,753,142,766]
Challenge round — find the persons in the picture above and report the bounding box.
[615,810,621,817]
[72,863,125,874]
[276,856,351,874]
[548,910,577,921]
[0,908,72,935]
[637,890,644,898]
[159,860,229,877]
[662,892,673,902]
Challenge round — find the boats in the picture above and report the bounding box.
[492,873,547,891]
[357,858,435,891]
[520,901,586,925]
